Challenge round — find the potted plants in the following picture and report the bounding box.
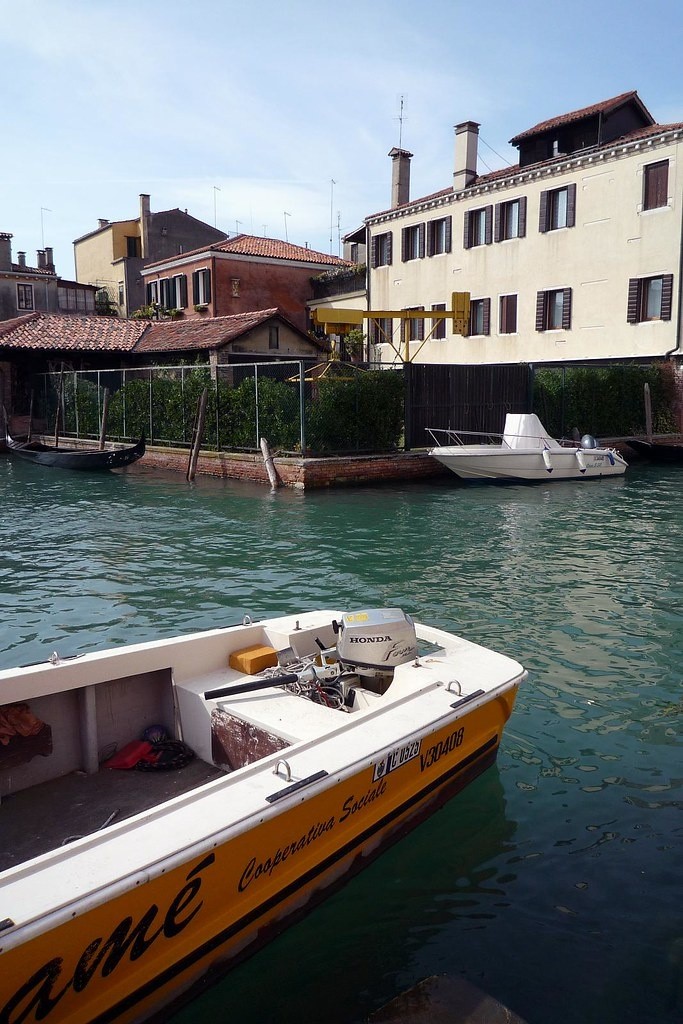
[345,329,366,362]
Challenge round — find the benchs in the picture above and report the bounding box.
[173,667,350,772]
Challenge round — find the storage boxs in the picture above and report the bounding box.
[228,643,280,675]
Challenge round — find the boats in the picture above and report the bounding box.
[5,428,147,470]
[425,411,628,482]
[0,602,532,1024]
